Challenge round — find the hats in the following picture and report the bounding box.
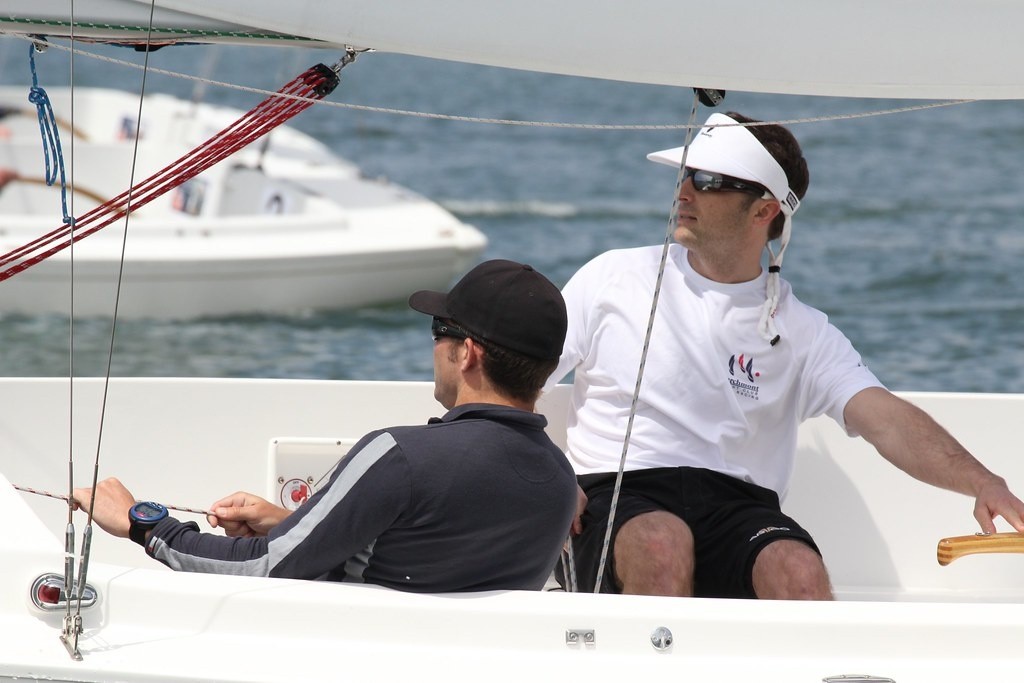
[409,258,567,360]
[646,113,801,216]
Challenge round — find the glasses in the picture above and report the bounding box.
[431,316,499,361]
[676,166,775,200]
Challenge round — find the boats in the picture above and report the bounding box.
[0,86,489,323]
[1,1,1023,681]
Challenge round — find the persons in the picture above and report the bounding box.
[535,109,1023,601]
[71,259,579,596]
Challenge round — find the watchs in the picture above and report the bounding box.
[127,501,170,547]
